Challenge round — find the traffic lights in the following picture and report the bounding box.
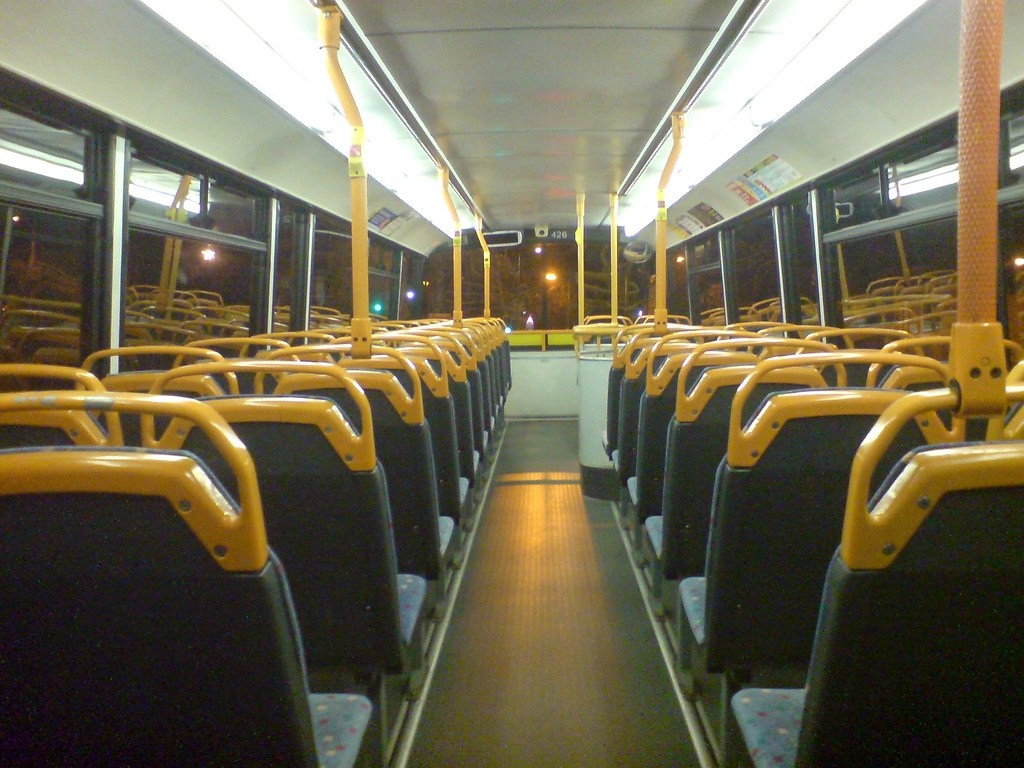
[371,295,384,315]
[405,285,417,304]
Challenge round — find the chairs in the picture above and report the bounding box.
[600,322,1024,768]
[0,260,387,365]
[0,316,512,768]
[637,271,1024,357]
